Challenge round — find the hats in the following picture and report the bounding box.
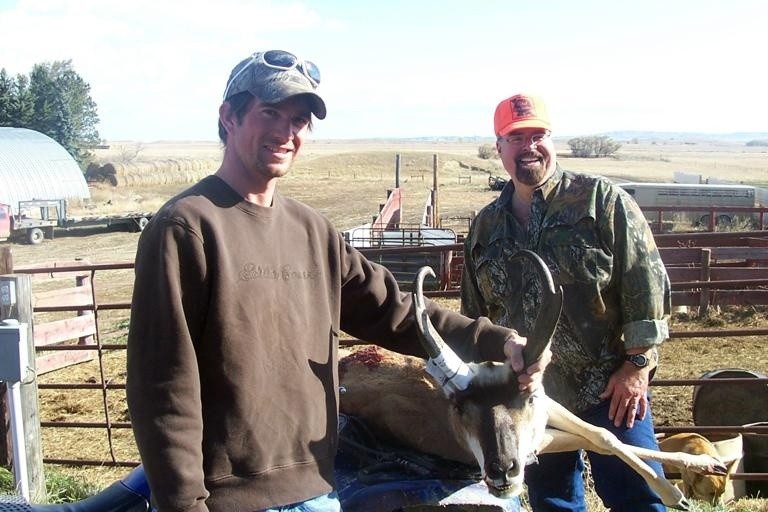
[493,94,554,137]
[222,50,327,120]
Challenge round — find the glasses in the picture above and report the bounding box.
[498,133,552,146]
[222,49,322,104]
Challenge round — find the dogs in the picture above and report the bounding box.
[657,431,738,506]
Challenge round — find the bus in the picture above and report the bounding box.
[608,178,756,233]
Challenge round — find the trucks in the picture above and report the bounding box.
[0,197,157,246]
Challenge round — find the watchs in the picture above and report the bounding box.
[622,352,660,370]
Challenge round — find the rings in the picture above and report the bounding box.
[630,402,638,406]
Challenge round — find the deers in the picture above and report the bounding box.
[338,249,730,512]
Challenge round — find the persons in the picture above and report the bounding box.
[460,91,674,512]
[121,47,553,512]
